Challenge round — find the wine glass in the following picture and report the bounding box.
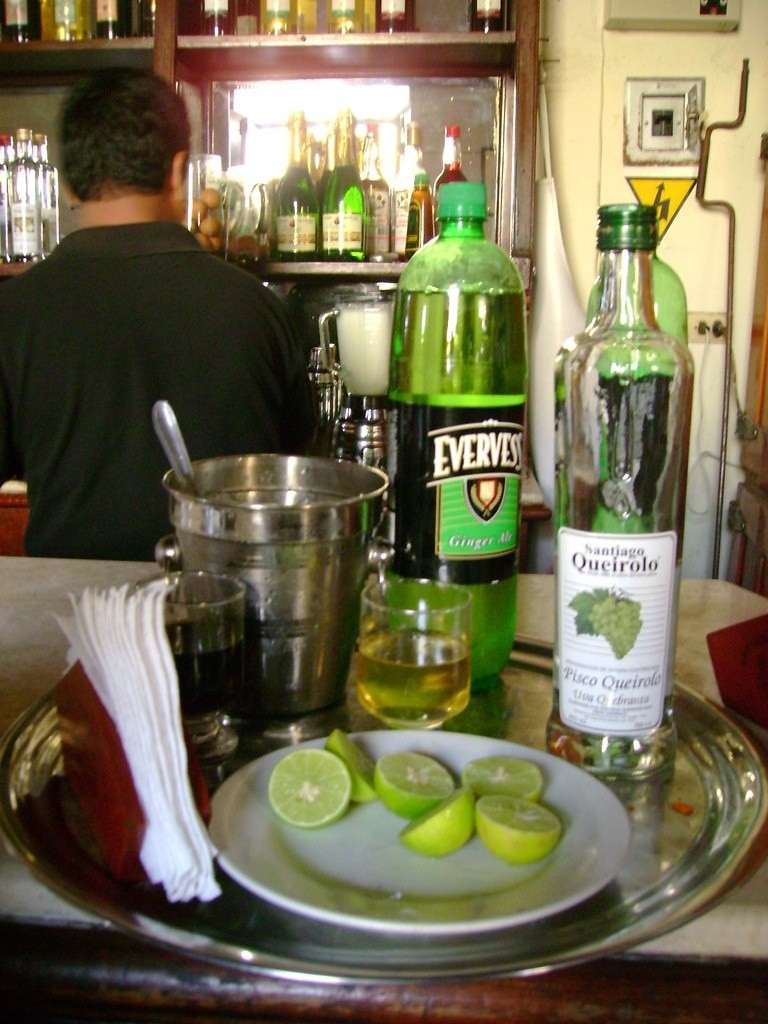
[130,570,250,760]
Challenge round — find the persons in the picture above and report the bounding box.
[0,65,316,562]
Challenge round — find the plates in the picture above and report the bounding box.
[198,730,627,934]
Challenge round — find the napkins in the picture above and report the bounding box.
[60,575,222,904]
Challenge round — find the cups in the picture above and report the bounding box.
[356,578,470,733]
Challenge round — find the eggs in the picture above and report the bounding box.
[186,188,222,252]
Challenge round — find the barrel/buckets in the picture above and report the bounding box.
[164,454,390,719]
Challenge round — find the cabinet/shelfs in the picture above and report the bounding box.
[0,0,553,573]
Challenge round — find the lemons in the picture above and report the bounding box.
[268,729,562,866]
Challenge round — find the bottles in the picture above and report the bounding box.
[0,128,60,265]
[184,109,472,261]
[1,0,157,41]
[548,205,697,779]
[390,183,529,692]
[183,0,508,40]
